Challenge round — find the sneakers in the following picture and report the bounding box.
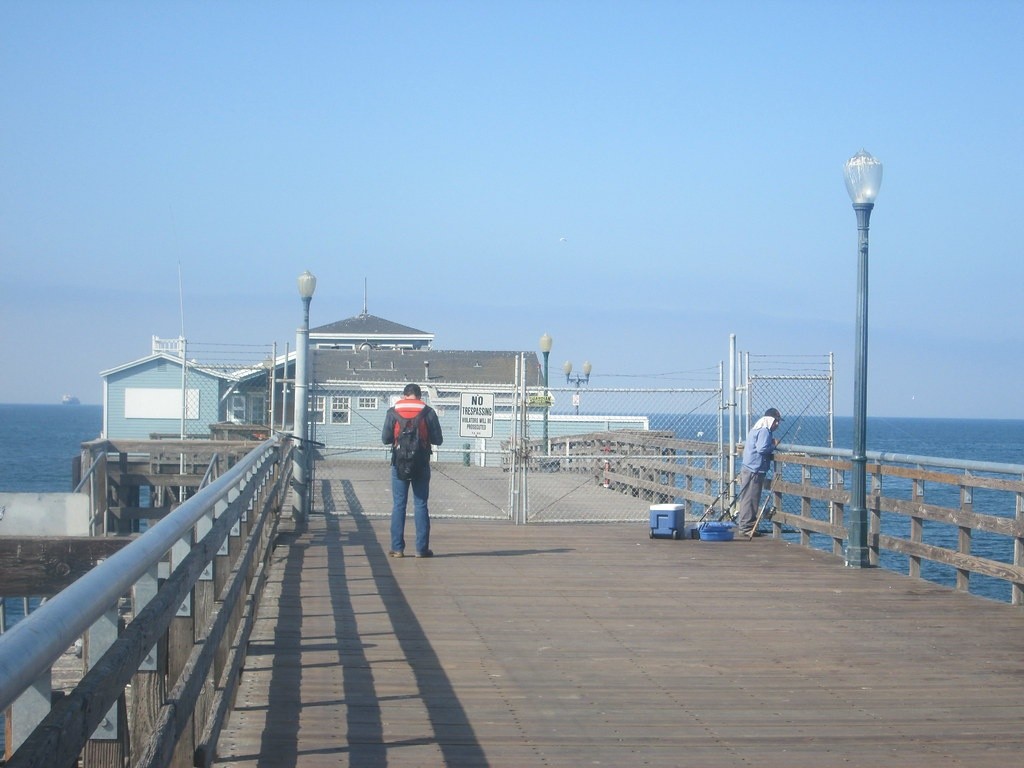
[388,550,404,558]
[415,550,433,558]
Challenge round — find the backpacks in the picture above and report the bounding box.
[389,405,432,482]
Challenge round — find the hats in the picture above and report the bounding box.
[764,408,784,421]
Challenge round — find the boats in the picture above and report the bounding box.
[62,395,80,405]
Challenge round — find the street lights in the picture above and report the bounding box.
[538,332,554,471]
[563,360,593,416]
[842,145,885,567]
[296,272,319,532]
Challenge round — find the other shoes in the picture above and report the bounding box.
[739,530,761,536]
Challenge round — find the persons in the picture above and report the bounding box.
[739,408,785,537]
[381,383,443,558]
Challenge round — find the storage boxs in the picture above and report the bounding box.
[649,504,685,539]
[698,522,737,541]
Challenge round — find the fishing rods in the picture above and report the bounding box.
[748,415,805,542]
[695,470,742,526]
[717,383,827,522]
